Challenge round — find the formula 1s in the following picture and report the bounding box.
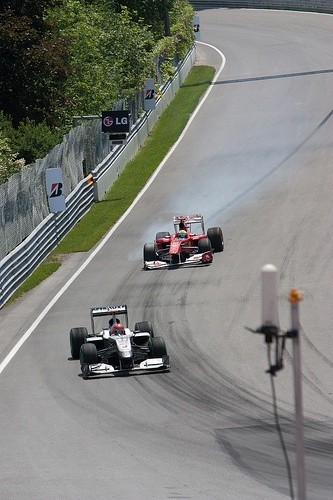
[142,214,225,271]
[69,304,171,380]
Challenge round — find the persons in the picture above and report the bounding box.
[110,323,125,336]
[178,229,188,240]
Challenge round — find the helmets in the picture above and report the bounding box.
[177,230,187,237]
[110,324,125,335]
